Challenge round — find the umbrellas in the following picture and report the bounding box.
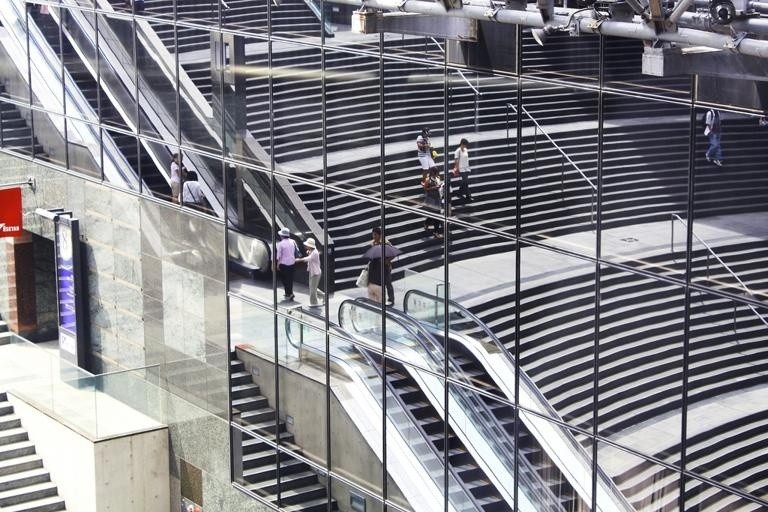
[364,245,403,261]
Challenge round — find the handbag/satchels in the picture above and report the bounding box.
[431,150,439,160]
[428,186,439,199]
[453,168,460,176]
[195,201,207,213]
[356,270,369,287]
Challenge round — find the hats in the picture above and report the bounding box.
[304,238,315,248]
[422,127,431,135]
[278,228,290,237]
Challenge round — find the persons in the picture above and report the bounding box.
[276,228,302,300]
[371,228,395,306]
[171,153,186,204]
[368,258,387,304]
[424,167,442,239]
[183,171,204,210]
[704,110,723,167]
[454,138,474,201]
[295,238,321,307]
[417,128,437,186]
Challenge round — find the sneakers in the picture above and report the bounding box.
[323,293,326,305]
[285,293,294,302]
[457,193,474,201]
[706,152,722,166]
[309,303,321,307]
[385,301,393,306]
[423,222,441,239]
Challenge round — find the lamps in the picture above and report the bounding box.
[351,4,384,35]
[529,4,608,48]
[640,39,687,79]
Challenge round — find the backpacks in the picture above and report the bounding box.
[702,111,713,127]
